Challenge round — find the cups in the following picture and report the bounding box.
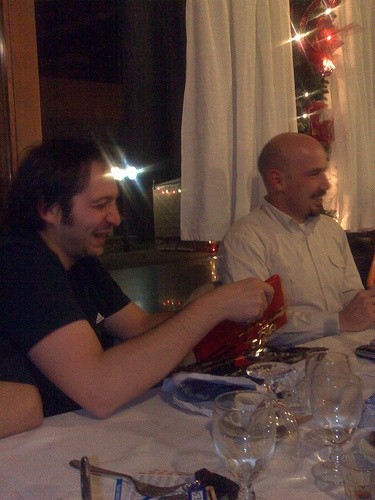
[229,393,268,426]
[339,452,375,500]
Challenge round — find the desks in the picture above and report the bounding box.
[0,328,375,500]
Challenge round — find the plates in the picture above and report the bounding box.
[220,417,280,437]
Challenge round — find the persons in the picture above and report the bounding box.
[214,132,375,350]
[0,137,274,438]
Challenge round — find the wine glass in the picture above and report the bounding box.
[212,391,276,500]
[246,362,301,475]
[305,351,362,482]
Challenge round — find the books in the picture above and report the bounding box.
[193,273,288,363]
